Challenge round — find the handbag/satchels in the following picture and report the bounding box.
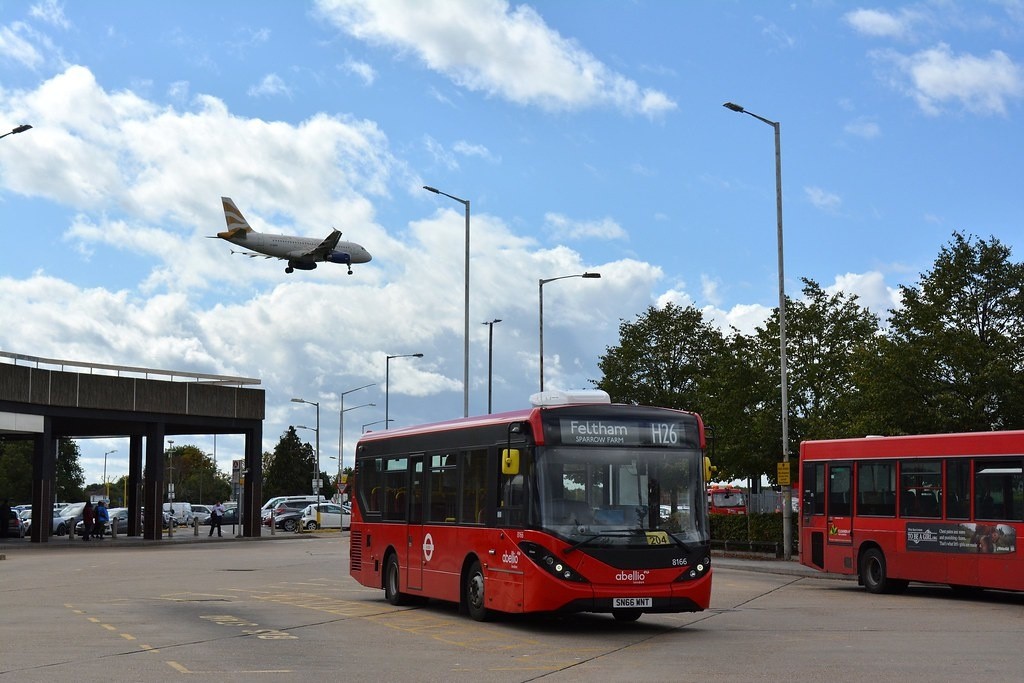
[100,512,106,518]
[211,511,217,520]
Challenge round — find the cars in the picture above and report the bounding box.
[275,504,351,531]
[20,509,66,536]
[142,506,179,527]
[203,507,238,525]
[75,507,145,535]
[660,503,691,519]
[262,511,271,526]
[192,504,214,524]
[298,503,351,531]
[10,506,30,518]
[9,509,28,538]
[59,501,99,533]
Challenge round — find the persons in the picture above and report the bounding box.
[82,500,109,541]
[208,501,225,537]
[971,523,1001,554]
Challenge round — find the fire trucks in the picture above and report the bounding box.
[706,482,748,515]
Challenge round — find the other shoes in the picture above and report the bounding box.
[82,537,91,541]
[208,534,212,537]
[218,535,223,537]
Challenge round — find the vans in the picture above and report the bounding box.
[162,502,196,528]
[16,502,71,509]
[261,495,327,520]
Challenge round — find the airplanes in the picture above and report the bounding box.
[206,195,372,274]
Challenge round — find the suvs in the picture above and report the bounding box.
[274,499,333,516]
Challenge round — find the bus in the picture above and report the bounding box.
[350,389,716,621]
[798,430,1024,594]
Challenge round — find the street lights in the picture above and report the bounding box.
[422,186,471,419]
[168,440,174,536]
[338,402,377,477]
[200,453,214,504]
[103,449,119,483]
[480,318,502,414]
[329,456,343,483]
[385,353,425,429]
[297,425,317,479]
[361,418,394,434]
[539,271,602,391]
[723,101,791,566]
[291,398,319,495]
